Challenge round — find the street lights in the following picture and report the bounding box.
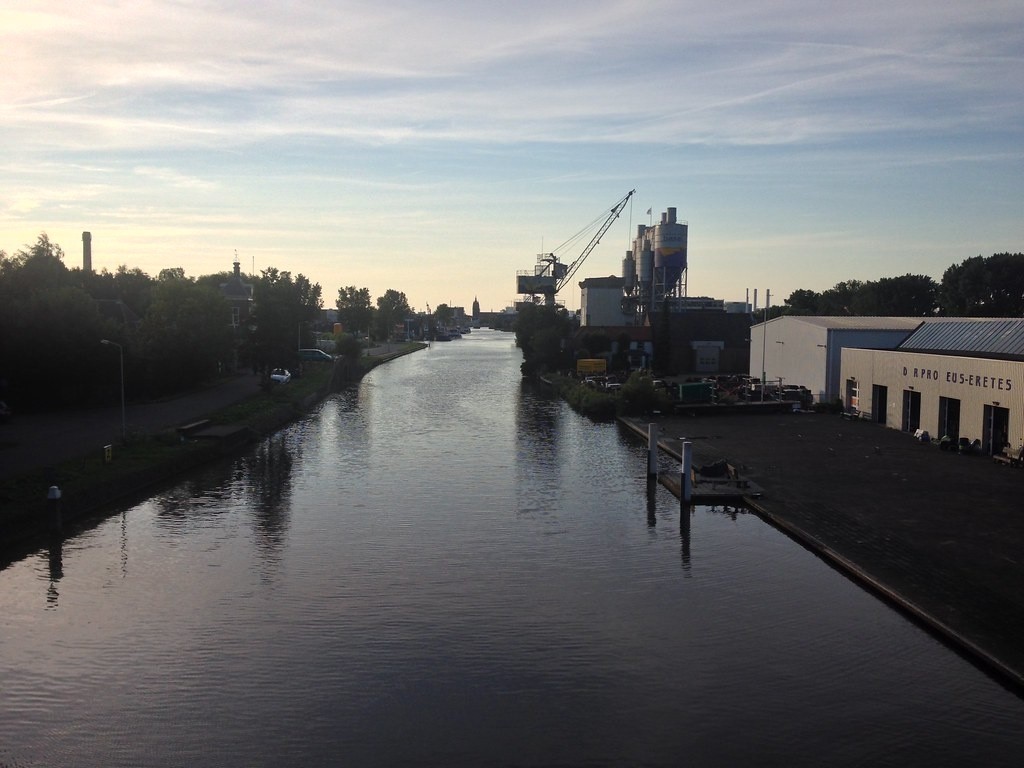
[298,320,309,375]
[100,338,127,446]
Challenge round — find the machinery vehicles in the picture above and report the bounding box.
[515,188,637,314]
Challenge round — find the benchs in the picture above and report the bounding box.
[842,410,862,420]
[993,447,1020,466]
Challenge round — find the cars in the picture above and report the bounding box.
[580,377,605,388]
[270,368,291,385]
